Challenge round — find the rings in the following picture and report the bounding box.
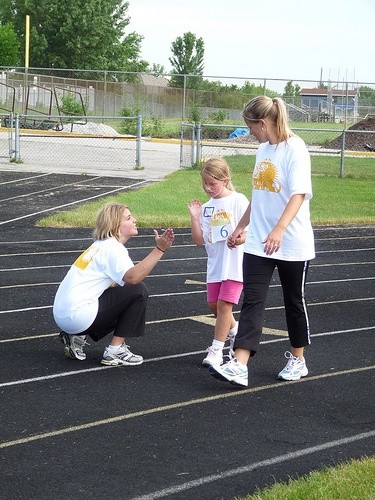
[275,245,277,246]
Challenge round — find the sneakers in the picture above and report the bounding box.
[226,334,236,360]
[208,358,249,387]
[277,351,310,381]
[100,341,144,366]
[200,345,223,366]
[58,330,91,360]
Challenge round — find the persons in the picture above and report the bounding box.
[53,201,174,367]
[209,95,317,388]
[186,157,251,367]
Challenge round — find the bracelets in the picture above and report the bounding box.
[156,246,166,253]
